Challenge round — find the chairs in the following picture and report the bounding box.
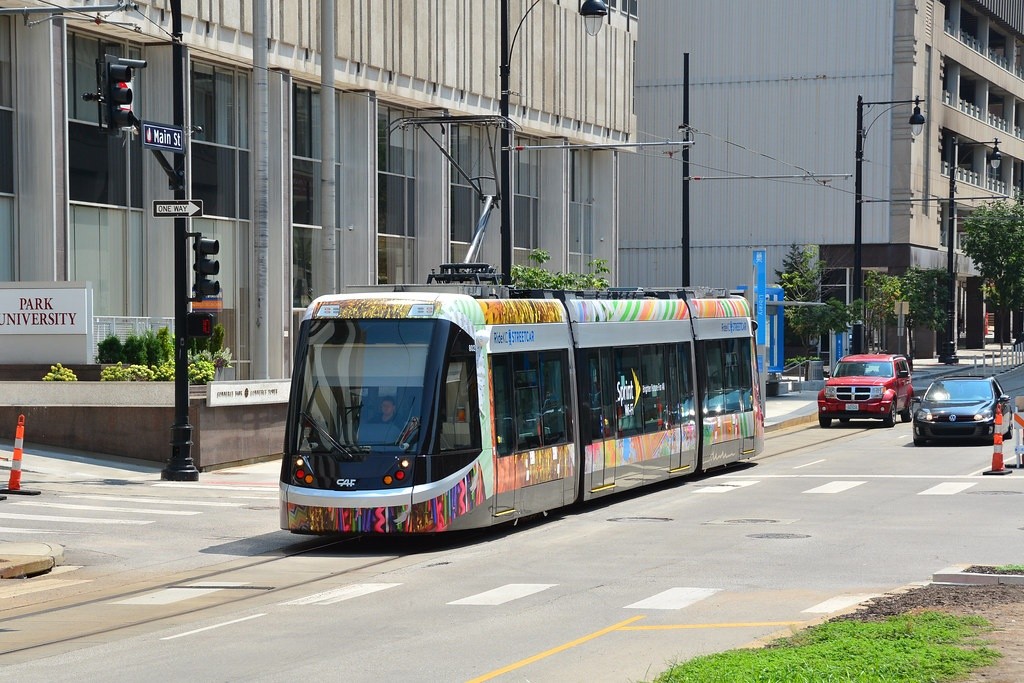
[857,366,865,376]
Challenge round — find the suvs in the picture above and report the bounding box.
[817,352,914,428]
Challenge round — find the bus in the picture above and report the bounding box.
[279,113,765,534]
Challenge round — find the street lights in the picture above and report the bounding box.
[945,135,1004,367]
[851,93,925,359]
[499,1,611,287]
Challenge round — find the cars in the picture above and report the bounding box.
[912,374,1015,446]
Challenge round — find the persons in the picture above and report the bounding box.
[362,396,404,444]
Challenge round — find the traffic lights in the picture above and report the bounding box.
[188,312,214,337]
[103,54,133,137]
[192,238,222,297]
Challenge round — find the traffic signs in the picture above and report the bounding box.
[155,200,205,219]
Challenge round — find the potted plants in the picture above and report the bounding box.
[805,356,824,380]
